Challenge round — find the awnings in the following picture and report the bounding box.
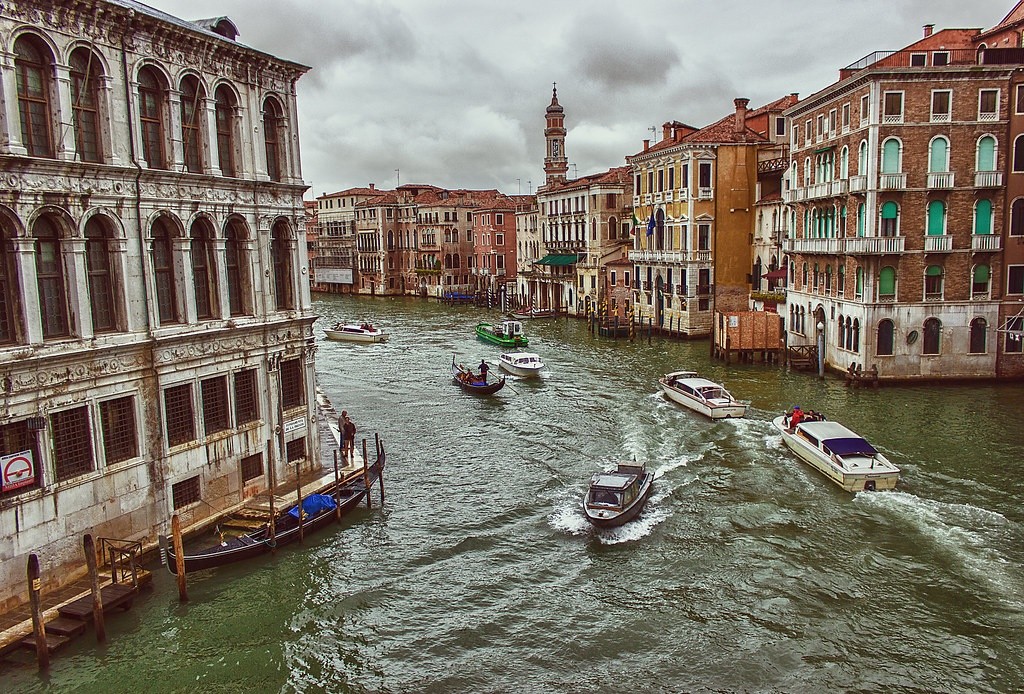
[813,144,838,155]
[532,252,588,267]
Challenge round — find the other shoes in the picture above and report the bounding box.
[340,447,354,458]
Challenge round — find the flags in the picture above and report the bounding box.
[645,209,657,237]
[629,213,637,235]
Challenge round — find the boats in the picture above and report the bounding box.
[583,455,657,529]
[508,294,555,321]
[448,354,506,395]
[167,430,388,575]
[658,370,749,419]
[320,323,390,344]
[773,410,902,496]
[473,319,529,348]
[498,351,545,379]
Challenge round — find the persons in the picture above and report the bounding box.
[509,328,514,339]
[478,358,490,384]
[603,491,619,504]
[343,417,356,458]
[360,323,364,328]
[338,410,351,452]
[668,375,678,386]
[808,409,818,421]
[364,322,369,330]
[464,368,474,384]
[369,324,374,332]
[783,405,804,428]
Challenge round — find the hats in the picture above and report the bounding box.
[793,406,800,409]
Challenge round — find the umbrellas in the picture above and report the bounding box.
[762,264,787,282]
